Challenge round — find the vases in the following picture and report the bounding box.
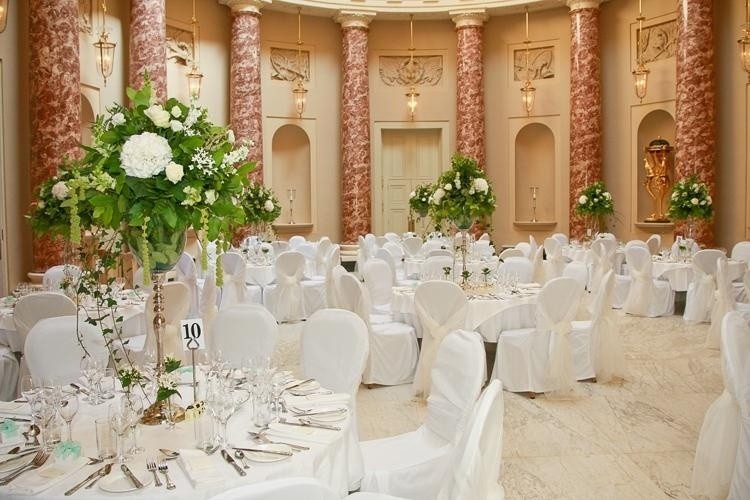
[686,217,697,239]
[592,212,600,235]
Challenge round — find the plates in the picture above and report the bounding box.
[241,442,293,467]
[308,407,351,423]
[285,380,321,397]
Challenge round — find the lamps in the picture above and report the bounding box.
[528,186,539,222]
[736,0,750,84]
[287,182,296,226]
[631,0,652,104]
[403,15,420,122]
[93,0,116,88]
[291,6,309,120]
[186,0,204,102]
[519,6,537,119]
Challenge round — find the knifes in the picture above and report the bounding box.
[69,382,91,396]
[121,462,143,490]
[221,448,246,480]
[285,378,317,393]
[277,421,343,431]
[64,462,115,494]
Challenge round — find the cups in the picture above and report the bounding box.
[38,402,64,447]
[98,365,117,402]
[193,410,216,449]
[250,353,281,427]
[94,417,117,459]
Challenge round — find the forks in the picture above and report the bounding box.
[157,460,176,490]
[147,454,160,486]
[3,447,49,487]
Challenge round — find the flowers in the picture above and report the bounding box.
[408,151,497,230]
[25,74,280,287]
[573,181,616,217]
[666,175,715,223]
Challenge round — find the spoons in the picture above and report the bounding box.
[235,450,252,473]
[86,463,111,490]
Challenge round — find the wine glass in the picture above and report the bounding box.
[21,373,78,447]
[80,355,104,408]
[196,349,256,405]
[120,395,147,453]
[418,266,522,293]
[13,277,126,309]
[211,392,237,447]
